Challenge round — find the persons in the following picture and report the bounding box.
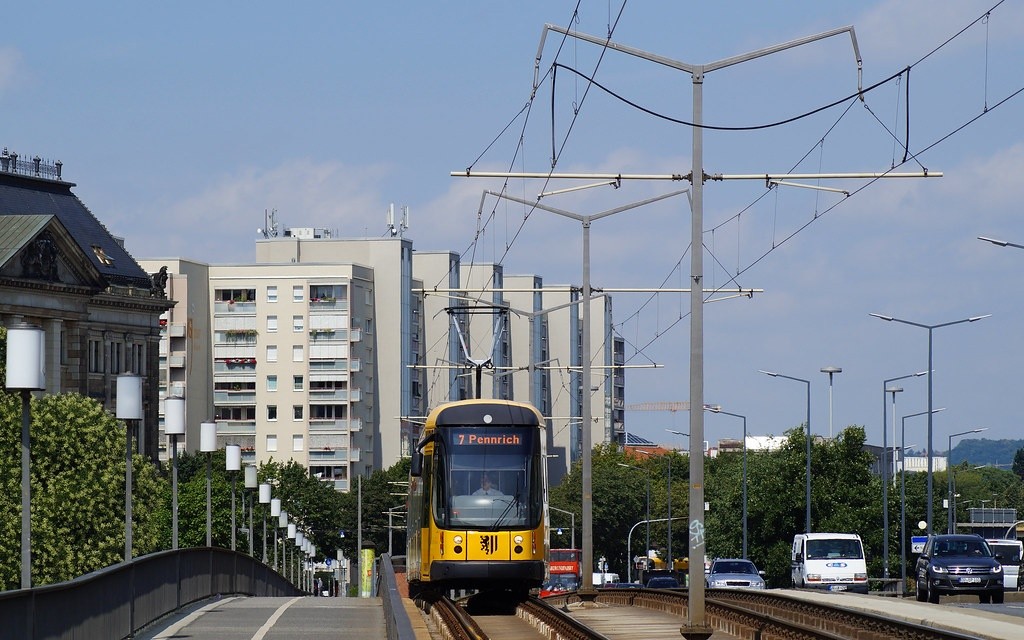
[472,473,505,496]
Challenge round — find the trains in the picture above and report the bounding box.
[403,300,549,611]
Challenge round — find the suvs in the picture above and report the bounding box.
[914,533,1004,605]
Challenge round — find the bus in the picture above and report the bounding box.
[542,550,584,599]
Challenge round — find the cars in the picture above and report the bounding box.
[703,558,766,589]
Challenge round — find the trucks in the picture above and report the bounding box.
[628,569,679,582]
[592,572,621,586]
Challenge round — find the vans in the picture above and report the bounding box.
[982,539,1024,591]
[792,533,871,594]
[635,555,684,572]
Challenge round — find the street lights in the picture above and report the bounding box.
[947,429,986,535]
[880,367,936,585]
[883,385,905,492]
[117,371,151,563]
[259,481,275,566]
[5,320,46,590]
[982,499,991,525]
[164,395,191,548]
[869,308,994,537]
[758,370,813,536]
[336,548,344,596]
[616,462,650,573]
[280,508,289,579]
[635,449,673,573]
[269,494,280,572]
[243,463,257,556]
[201,418,218,546]
[881,445,920,485]
[709,409,748,558]
[226,441,242,550]
[287,522,316,595]
[900,407,951,590]
[992,493,1000,526]
[821,367,844,442]
[952,465,985,537]
